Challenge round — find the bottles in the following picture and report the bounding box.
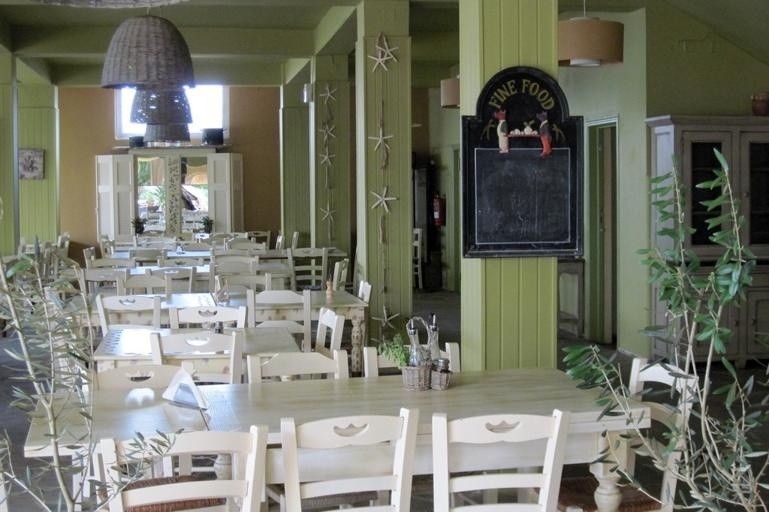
[407,318,425,366]
[436,358,451,374]
[425,315,442,366]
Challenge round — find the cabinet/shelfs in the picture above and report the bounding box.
[651,265,766,367]
[645,111,769,263]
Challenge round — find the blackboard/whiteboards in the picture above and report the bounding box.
[470,143,577,249]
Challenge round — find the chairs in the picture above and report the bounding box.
[10,233,702,502]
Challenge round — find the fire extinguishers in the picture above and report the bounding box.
[433,191,444,225]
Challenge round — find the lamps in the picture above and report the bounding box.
[100,0,195,145]
[440,72,467,110]
[557,0,629,70]
